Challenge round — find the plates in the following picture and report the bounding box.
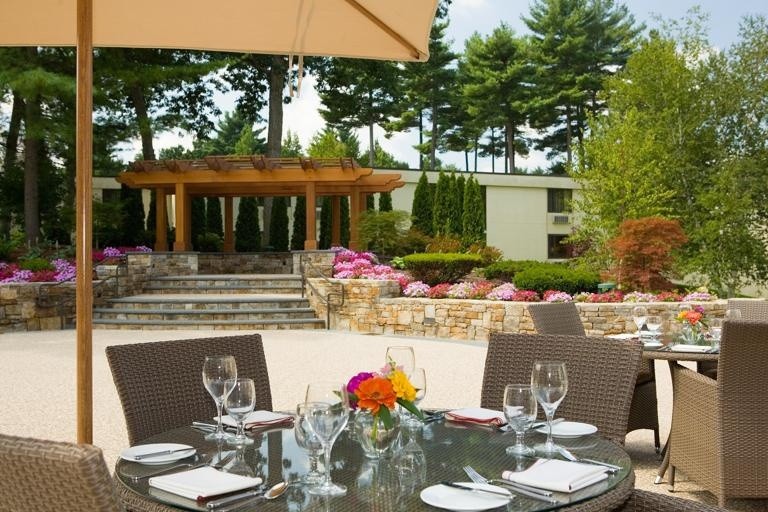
[420,481,513,511]
[533,419,598,439]
[120,443,197,466]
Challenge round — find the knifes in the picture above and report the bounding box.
[133,447,197,460]
[441,480,516,499]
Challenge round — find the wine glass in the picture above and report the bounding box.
[202,356,236,440]
[385,346,416,424]
[503,383,537,456]
[406,367,427,427]
[394,420,428,494]
[646,310,662,343]
[709,318,722,350]
[632,307,647,342]
[306,383,349,498]
[223,379,257,446]
[296,403,330,486]
[529,360,569,454]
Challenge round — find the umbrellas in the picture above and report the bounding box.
[0,0,440,443]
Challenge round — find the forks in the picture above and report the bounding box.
[187,452,237,469]
[462,464,558,503]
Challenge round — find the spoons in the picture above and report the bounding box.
[217,480,289,512]
[558,445,624,470]
[134,453,212,480]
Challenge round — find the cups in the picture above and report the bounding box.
[725,309,742,319]
